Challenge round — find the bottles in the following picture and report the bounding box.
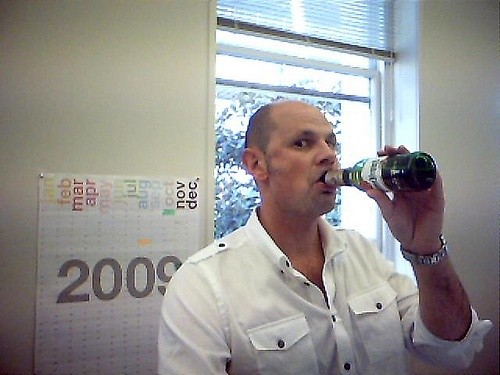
[321,152,437,192]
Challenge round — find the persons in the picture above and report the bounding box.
[156,99,494,375]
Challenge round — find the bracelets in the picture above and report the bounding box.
[400,232,449,265]
